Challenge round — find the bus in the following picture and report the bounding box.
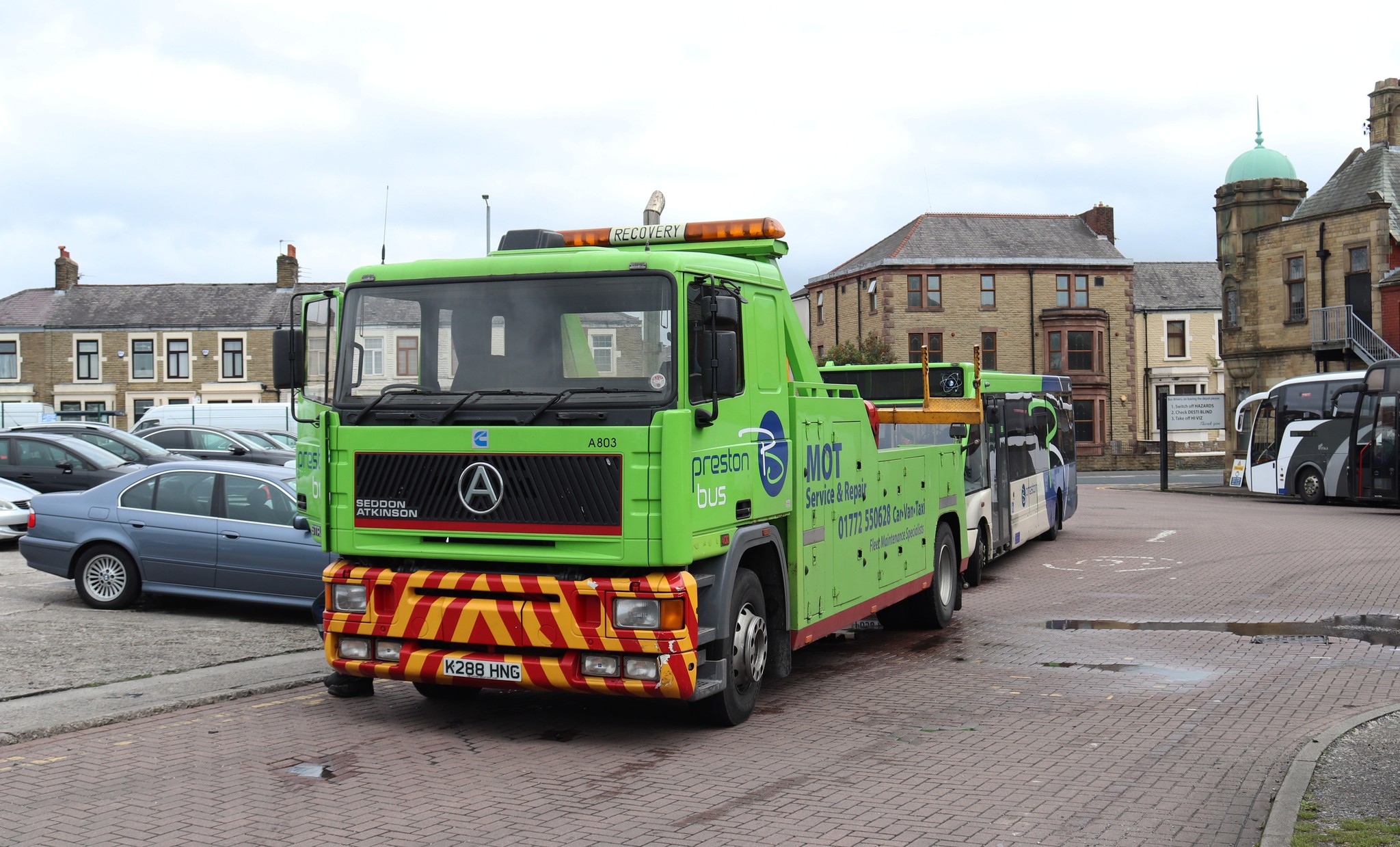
[819,360,1079,587]
[1235,370,1394,506]
[1329,358,1400,504]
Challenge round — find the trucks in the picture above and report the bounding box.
[272,189,968,729]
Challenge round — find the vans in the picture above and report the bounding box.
[127,402,298,447]
[0,402,56,431]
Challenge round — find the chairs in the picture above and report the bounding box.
[452,353,552,392]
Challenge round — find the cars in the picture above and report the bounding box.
[17,461,339,611]
[0,420,299,512]
[0,477,43,543]
